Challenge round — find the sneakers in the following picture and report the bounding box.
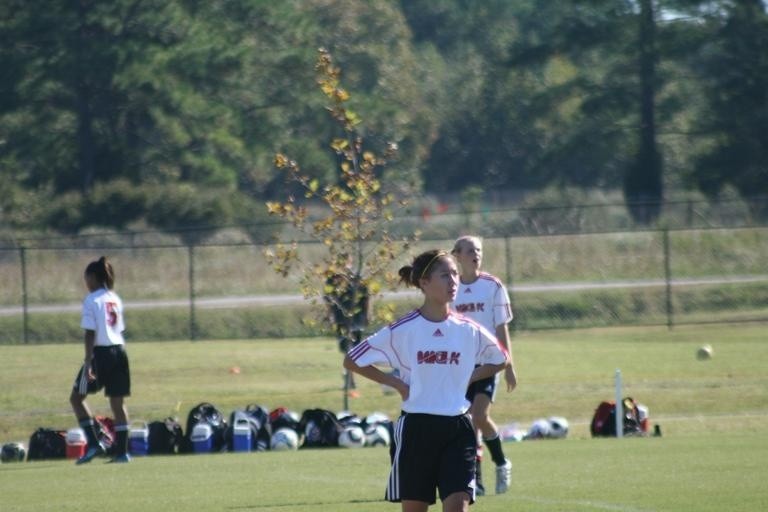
[495,458,512,494]
[475,483,485,496]
[77,441,105,463]
[108,453,131,462]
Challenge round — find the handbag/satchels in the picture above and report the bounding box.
[591,397,649,435]
[94,415,115,454]
[229,405,343,447]
[148,417,193,453]
[29,430,66,459]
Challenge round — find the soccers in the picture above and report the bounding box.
[694,345,711,361]
[268,411,391,449]
[530,416,567,438]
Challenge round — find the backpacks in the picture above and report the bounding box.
[187,403,227,449]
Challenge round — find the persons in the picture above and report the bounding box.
[441,237,518,494]
[341,251,511,512]
[70,255,130,464]
[324,254,368,397]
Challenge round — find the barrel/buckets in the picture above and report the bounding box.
[65,430,85,458]
[233,416,251,451]
[129,419,149,455]
[190,423,213,451]
